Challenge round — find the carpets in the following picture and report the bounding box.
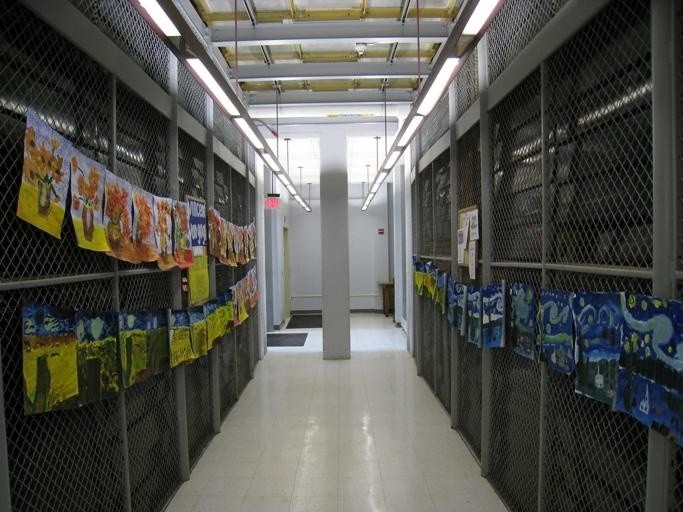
[286,315,322,329]
[267,333,308,346]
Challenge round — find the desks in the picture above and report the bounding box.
[379,282,393,317]
